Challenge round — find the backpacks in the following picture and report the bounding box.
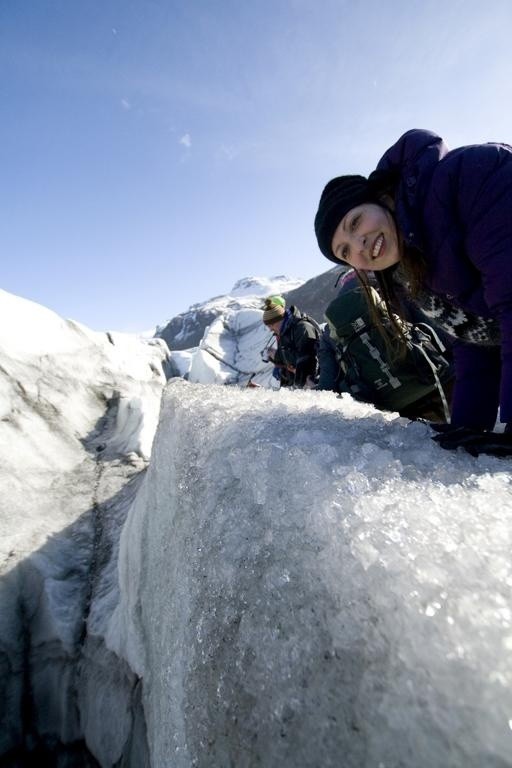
[325,286,449,412]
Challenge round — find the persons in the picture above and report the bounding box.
[259,268,455,427]
[313,128,512,458]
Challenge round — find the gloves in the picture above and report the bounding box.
[430,423,512,458]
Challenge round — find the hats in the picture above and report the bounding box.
[263,295,286,325]
[315,175,379,267]
